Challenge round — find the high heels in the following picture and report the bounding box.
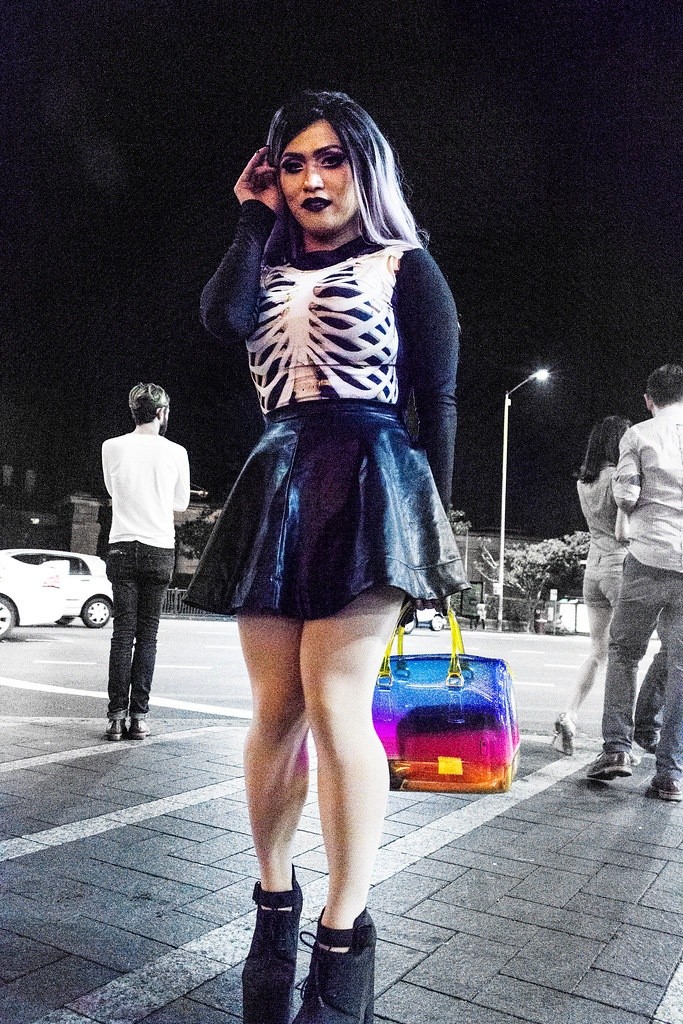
[291,906,377,1024]
[241,863,303,1004]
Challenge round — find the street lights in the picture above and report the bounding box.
[497,369,549,633]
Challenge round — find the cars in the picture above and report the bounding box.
[0,549,113,640]
[415,608,448,631]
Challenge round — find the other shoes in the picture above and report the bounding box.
[629,754,642,768]
[555,713,575,754]
[634,733,659,755]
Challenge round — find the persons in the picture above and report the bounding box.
[100,381,190,739]
[631,622,669,753]
[585,362,683,801]
[545,414,636,754]
[181,88,451,1024]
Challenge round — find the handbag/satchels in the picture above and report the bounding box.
[372,598,520,792]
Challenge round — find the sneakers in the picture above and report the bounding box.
[105,718,128,741]
[586,751,633,781]
[128,717,151,740]
[652,776,683,801]
